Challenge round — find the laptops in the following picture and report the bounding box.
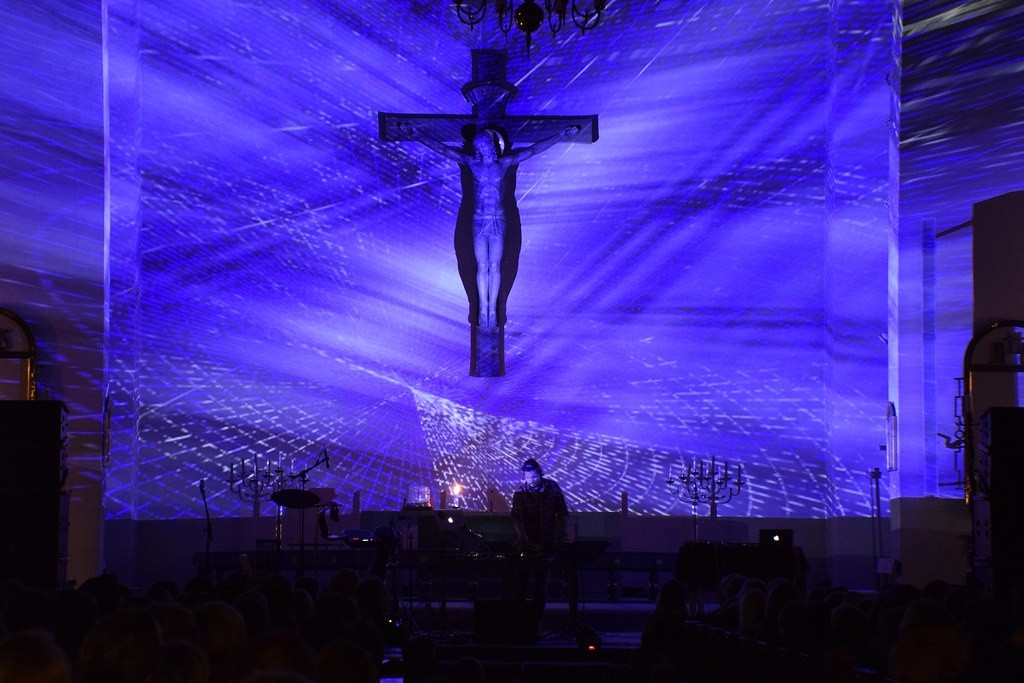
[758,528,793,549]
[434,509,482,538]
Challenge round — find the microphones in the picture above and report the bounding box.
[323,447,329,469]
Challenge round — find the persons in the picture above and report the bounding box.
[0,568,381,683]
[399,122,579,330]
[511,459,574,548]
[642,572,1024,683]
